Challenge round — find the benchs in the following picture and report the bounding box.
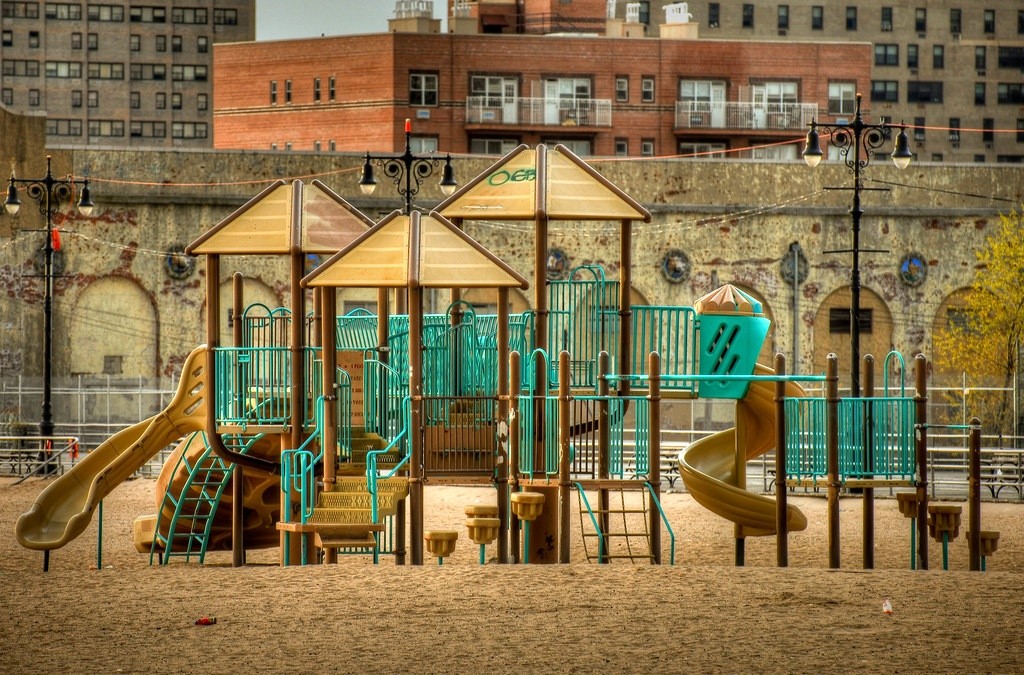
[0,452,37,474]
[967,452,1024,499]
[626,449,681,488]
[766,464,826,493]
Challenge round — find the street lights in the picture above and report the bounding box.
[356,110,458,216]
[802,93,914,493]
[4,154,94,474]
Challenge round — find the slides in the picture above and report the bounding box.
[16,404,208,549]
[677,364,809,534]
